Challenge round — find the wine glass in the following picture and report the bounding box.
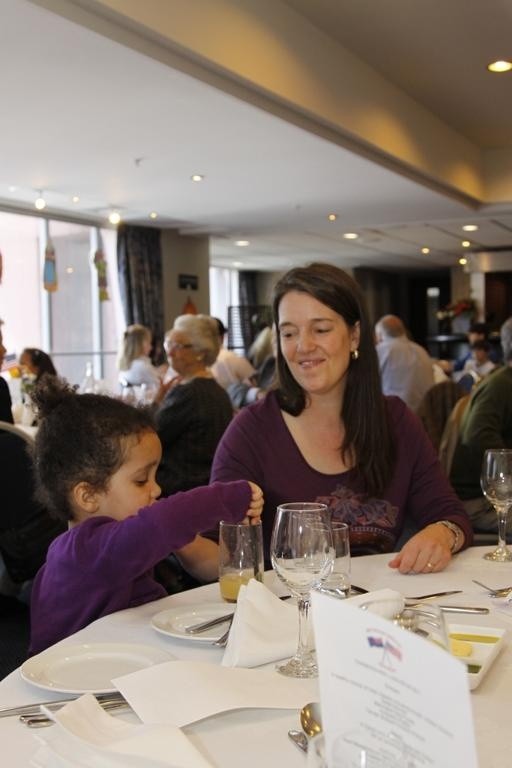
[359,600,450,653]
[270,503,334,676]
[480,449,512,561]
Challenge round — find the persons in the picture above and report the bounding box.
[154,313,234,500]
[209,262,473,576]
[27,394,264,659]
[17,348,57,376]
[0,317,15,425]
[118,324,162,386]
[162,318,255,390]
[438,314,512,538]
[373,314,435,412]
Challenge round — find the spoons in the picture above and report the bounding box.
[473,579,512,598]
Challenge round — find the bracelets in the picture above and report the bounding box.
[436,520,460,553]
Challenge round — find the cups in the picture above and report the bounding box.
[306,727,409,768]
[218,517,266,602]
[305,522,350,599]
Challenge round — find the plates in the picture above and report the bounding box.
[151,602,237,642]
[22,642,176,694]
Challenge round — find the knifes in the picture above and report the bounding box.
[184,593,293,636]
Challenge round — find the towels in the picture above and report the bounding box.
[35,702,174,768]
[217,578,298,669]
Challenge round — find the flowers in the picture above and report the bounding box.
[437,312,454,322]
[447,299,473,319]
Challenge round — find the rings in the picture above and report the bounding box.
[427,562,436,568]
